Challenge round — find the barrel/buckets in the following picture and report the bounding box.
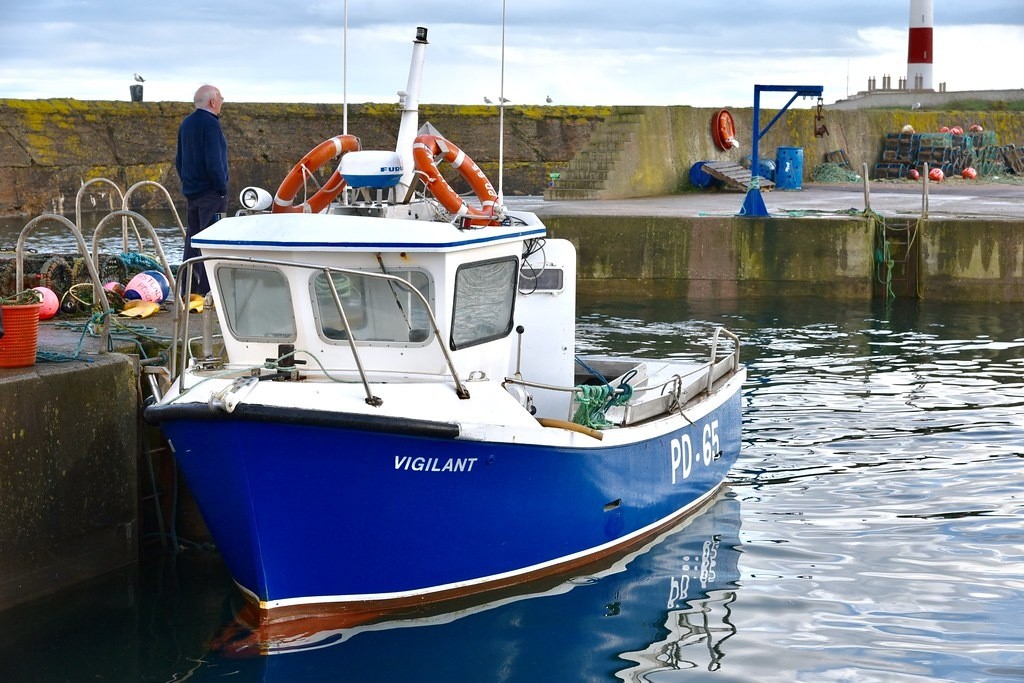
[690,161,727,189]
[0,300,45,369]
[752,160,775,180]
[774,147,803,189]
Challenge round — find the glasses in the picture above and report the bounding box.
[218,97,225,102]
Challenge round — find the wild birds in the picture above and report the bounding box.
[132,72,146,85]
[483,96,493,105]
[498,96,511,105]
[545,95,554,105]
[912,102,921,112]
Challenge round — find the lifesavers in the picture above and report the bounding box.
[266,133,366,220]
[720,112,734,145]
[407,134,509,230]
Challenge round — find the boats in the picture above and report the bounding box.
[141,1,749,654]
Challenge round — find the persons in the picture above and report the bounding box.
[175,85,229,297]
